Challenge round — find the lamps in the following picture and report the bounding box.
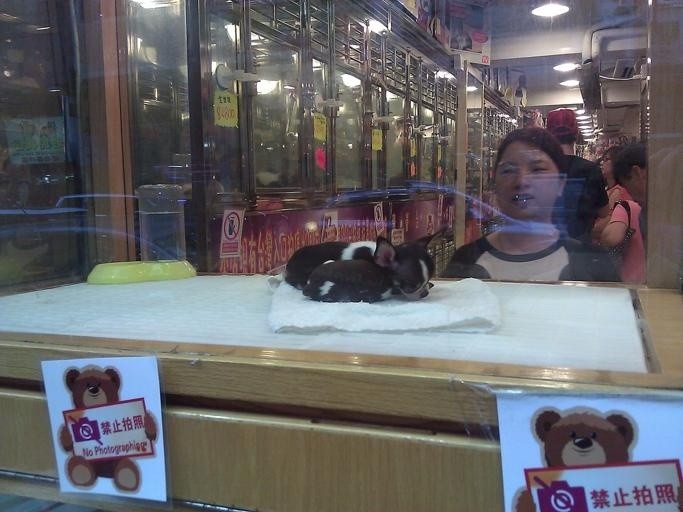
[214,64,261,90]
[313,94,344,113]
[414,126,498,156]
[372,113,394,127]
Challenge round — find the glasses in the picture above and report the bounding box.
[597,154,613,163]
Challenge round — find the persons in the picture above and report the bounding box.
[437,126,623,284]
[545,107,611,240]
[591,144,633,243]
[600,198,646,286]
[199,147,409,210]
[609,142,649,249]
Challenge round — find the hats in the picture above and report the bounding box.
[613,143,645,165]
[544,107,579,138]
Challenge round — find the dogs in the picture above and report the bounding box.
[284,225,450,304]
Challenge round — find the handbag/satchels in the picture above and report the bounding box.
[573,241,618,281]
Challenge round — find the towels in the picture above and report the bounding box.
[268,279,504,332]
